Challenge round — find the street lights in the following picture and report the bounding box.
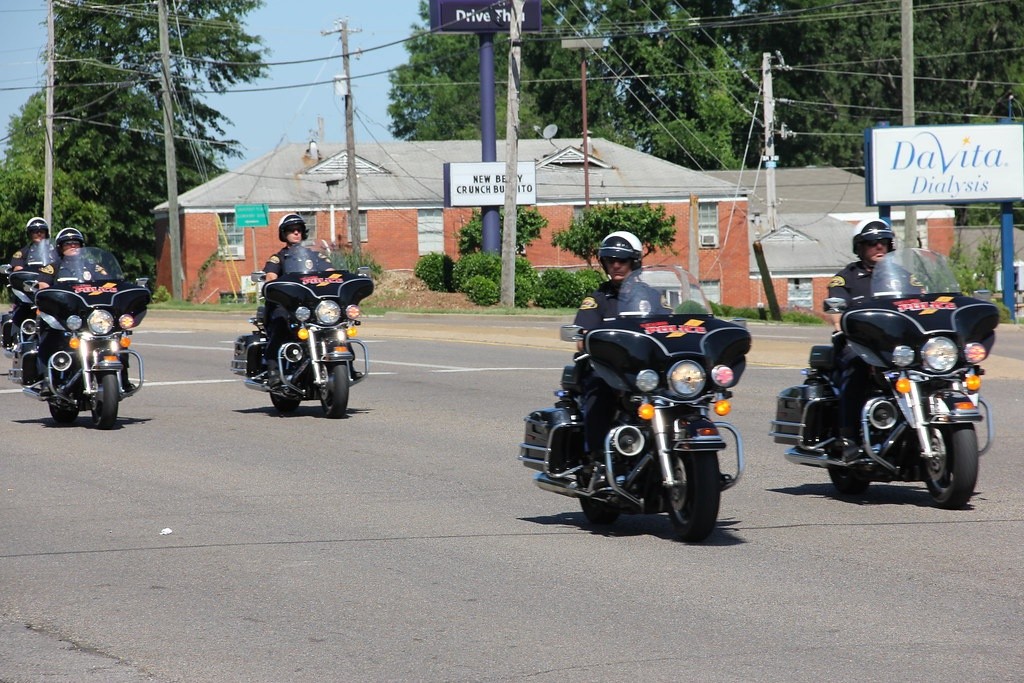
[560,37,605,266]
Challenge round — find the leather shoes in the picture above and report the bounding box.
[41,384,51,396]
[131,391,132,391]
[351,370,363,380]
[825,446,863,463]
[720,473,732,488]
[269,370,281,389]
[123,381,136,394]
[576,468,604,490]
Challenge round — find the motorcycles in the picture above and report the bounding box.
[1,238,61,359]
[519,260,754,542]
[9,245,151,430]
[227,238,376,421]
[776,245,1002,509]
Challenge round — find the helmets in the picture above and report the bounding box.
[26,217,51,239]
[277,213,307,242]
[56,228,85,257]
[852,218,894,252]
[597,231,645,271]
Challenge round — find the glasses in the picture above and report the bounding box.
[862,236,892,248]
[605,256,630,265]
[286,226,303,233]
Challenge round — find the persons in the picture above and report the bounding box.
[2,216,59,353]
[263,213,366,389]
[24,227,144,398]
[574,230,735,490]
[821,218,928,462]
